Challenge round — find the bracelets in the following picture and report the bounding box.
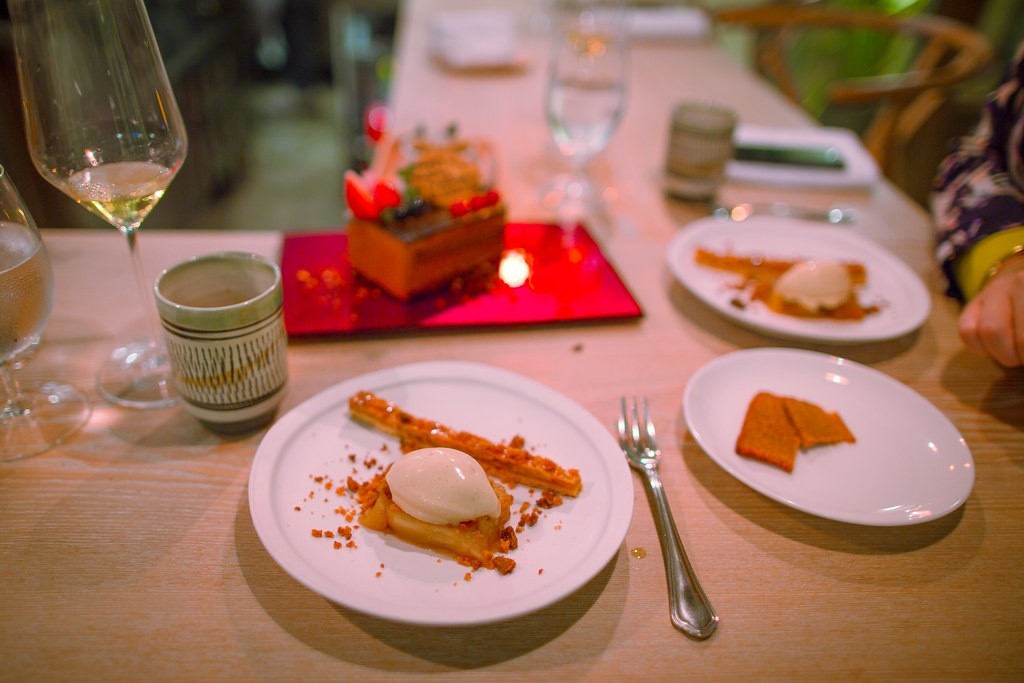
[979,242,1024,292]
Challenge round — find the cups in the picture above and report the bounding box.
[664,99,736,204]
[153,248,292,436]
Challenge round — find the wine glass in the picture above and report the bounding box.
[0,167,89,465]
[541,23,631,224]
[9,1,188,413]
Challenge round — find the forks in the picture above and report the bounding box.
[617,390,719,641]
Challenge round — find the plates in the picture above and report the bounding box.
[276,221,643,343]
[727,124,879,192]
[668,218,930,345]
[245,359,638,625]
[615,10,713,40]
[681,347,974,529]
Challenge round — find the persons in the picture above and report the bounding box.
[924,34,1024,374]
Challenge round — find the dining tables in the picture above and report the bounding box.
[1,228,1024,682]
[371,1,936,228]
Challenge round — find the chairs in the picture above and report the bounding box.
[713,8,995,193]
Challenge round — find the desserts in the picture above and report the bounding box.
[295,390,580,579]
[345,121,507,302]
[736,392,859,472]
[700,244,886,325]
